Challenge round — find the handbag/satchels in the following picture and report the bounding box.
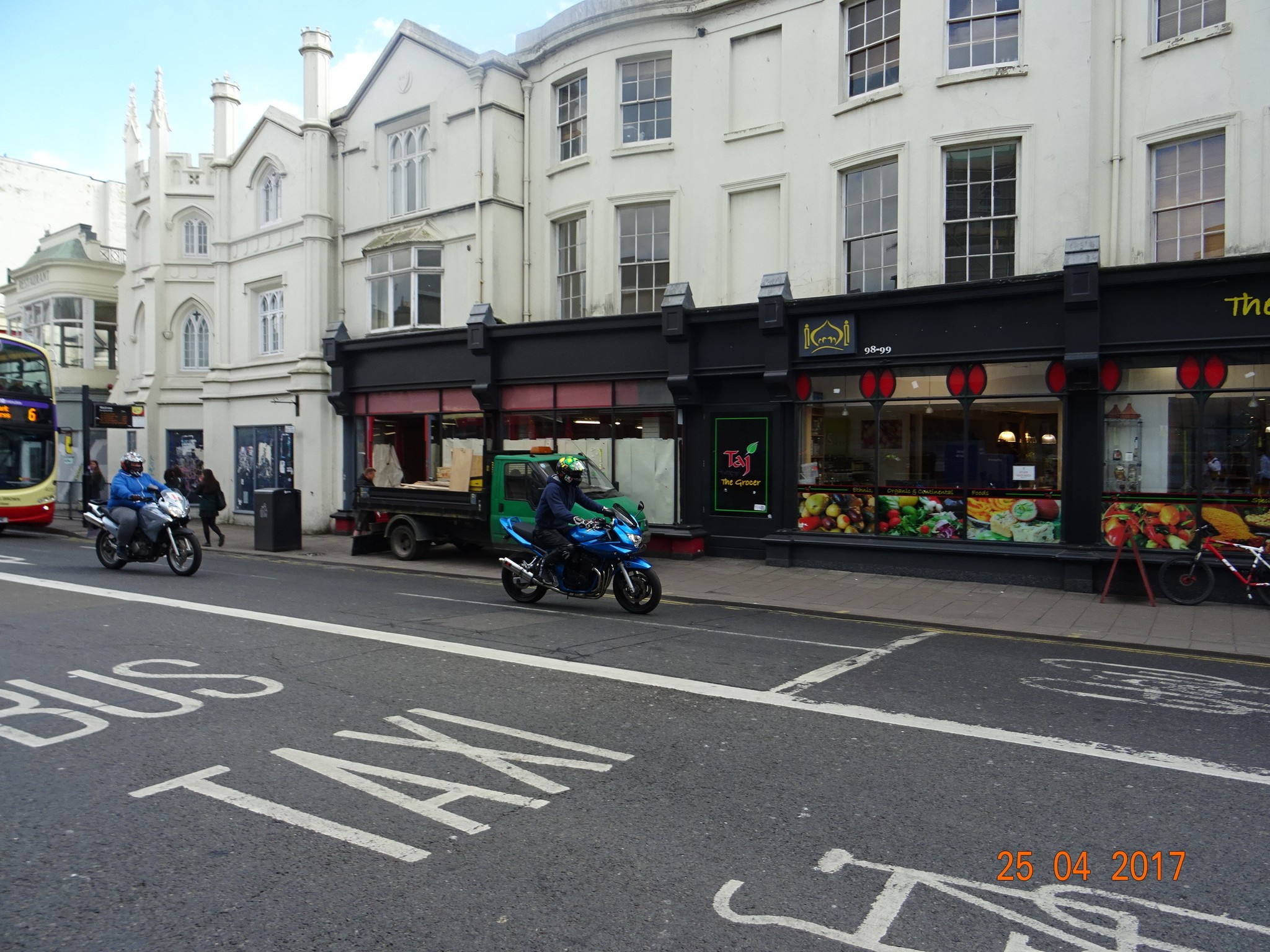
[216,490,227,511]
[99,476,105,490]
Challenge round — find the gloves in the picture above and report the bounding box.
[573,516,595,530]
[602,506,609,514]
[130,494,140,501]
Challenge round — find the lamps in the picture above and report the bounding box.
[1042,427,1056,444]
[925,376,934,413]
[997,424,1015,442]
[1248,365,1258,407]
[841,376,849,415]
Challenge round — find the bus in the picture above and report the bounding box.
[0,332,74,529]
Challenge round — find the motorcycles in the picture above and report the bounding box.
[82,481,202,576]
[498,501,662,614]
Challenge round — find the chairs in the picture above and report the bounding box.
[508,469,530,501]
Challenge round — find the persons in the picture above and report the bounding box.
[353,468,379,537]
[166,468,226,547]
[1200,447,1270,499]
[108,451,177,561]
[89,459,106,528]
[535,457,612,591]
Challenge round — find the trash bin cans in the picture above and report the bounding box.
[253,487,302,552]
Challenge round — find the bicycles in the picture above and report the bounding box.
[1157,524,1270,608]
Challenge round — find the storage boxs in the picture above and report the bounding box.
[801,462,818,478]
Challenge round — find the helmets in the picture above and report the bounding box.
[120,451,146,478]
[556,456,588,486]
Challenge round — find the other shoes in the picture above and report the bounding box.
[202,542,211,547]
[218,534,224,546]
[535,558,552,584]
[116,545,127,559]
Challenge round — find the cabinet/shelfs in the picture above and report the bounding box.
[1104,418,1143,492]
[1172,452,1255,493]
[804,406,825,476]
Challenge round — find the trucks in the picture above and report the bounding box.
[354,446,652,562]
